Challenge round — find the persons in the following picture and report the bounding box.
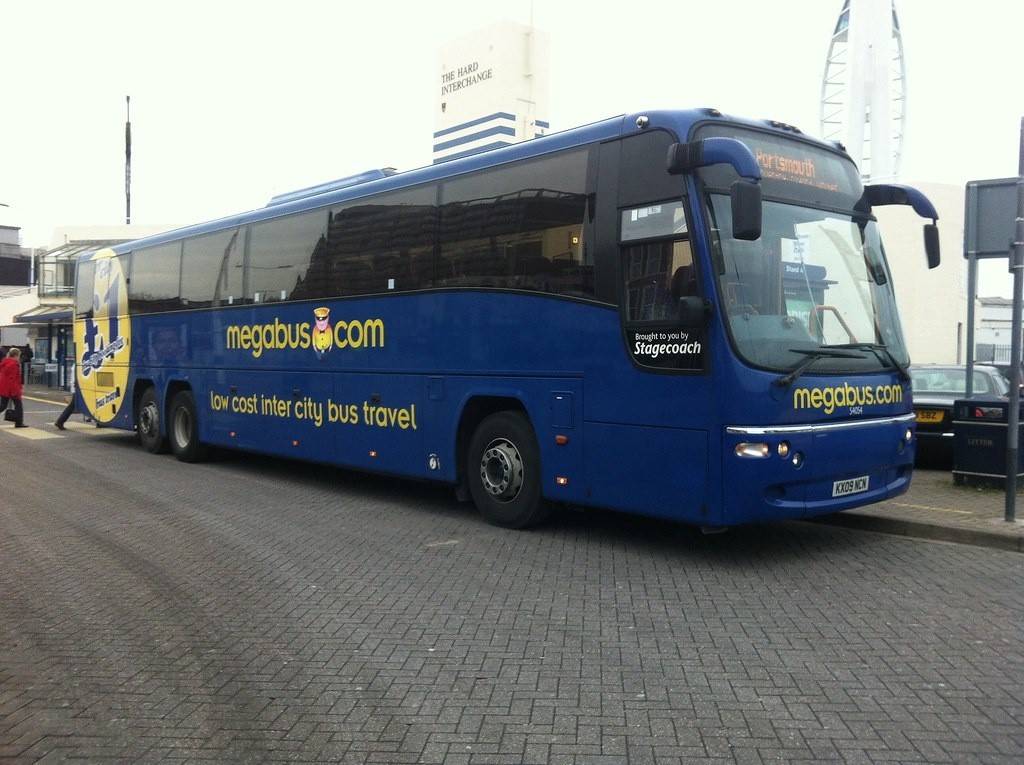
[21,343,33,384]
[0,347,29,429]
[54,363,110,430]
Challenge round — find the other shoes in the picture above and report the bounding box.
[55,422,66,430]
[96,423,102,428]
[15,422,28,428]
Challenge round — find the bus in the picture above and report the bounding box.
[72,108,941,530]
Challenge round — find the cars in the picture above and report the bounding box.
[904,366,1013,466]
[970,360,1024,392]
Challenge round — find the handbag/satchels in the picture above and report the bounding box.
[4,398,16,422]
